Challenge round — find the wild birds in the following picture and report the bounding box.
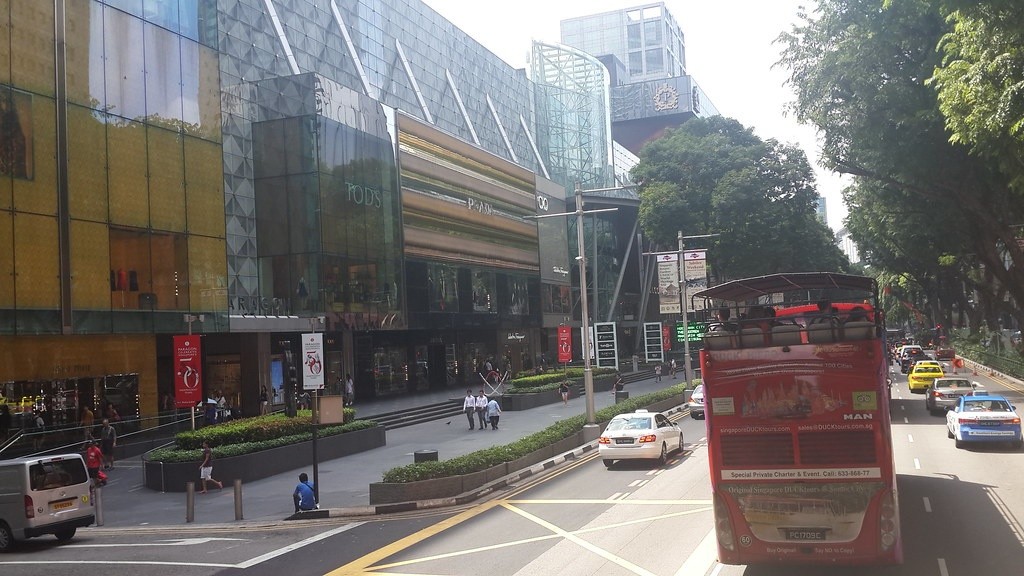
[446,421,451,425]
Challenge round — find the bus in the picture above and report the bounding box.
[690,270,907,568]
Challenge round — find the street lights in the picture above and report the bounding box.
[641,229,721,392]
[519,182,638,426]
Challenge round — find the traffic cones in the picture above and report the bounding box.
[952,363,960,375]
[972,364,979,377]
[988,366,996,377]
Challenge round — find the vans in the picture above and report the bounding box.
[0,453,98,554]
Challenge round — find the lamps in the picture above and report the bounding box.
[318,317,325,324]
[199,315,205,323]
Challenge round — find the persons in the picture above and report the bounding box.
[485,362,492,374]
[558,383,568,405]
[261,385,269,415]
[100,419,116,472]
[708,306,866,334]
[671,358,677,379]
[86,441,104,488]
[655,362,662,383]
[33,410,45,451]
[463,390,502,430]
[293,473,316,513]
[107,404,120,437]
[162,390,226,426]
[199,440,223,494]
[614,374,625,391]
[79,406,98,451]
[345,374,354,405]
[503,360,511,379]
[300,390,311,410]
[297,275,308,305]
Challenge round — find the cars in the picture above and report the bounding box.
[894,341,947,393]
[689,383,705,420]
[943,390,1023,449]
[934,344,955,360]
[598,409,685,465]
[923,377,978,414]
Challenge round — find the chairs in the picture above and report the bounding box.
[640,423,648,429]
[918,369,923,372]
[931,369,938,372]
[991,403,1000,411]
[701,312,877,351]
[959,382,968,387]
[940,382,949,387]
